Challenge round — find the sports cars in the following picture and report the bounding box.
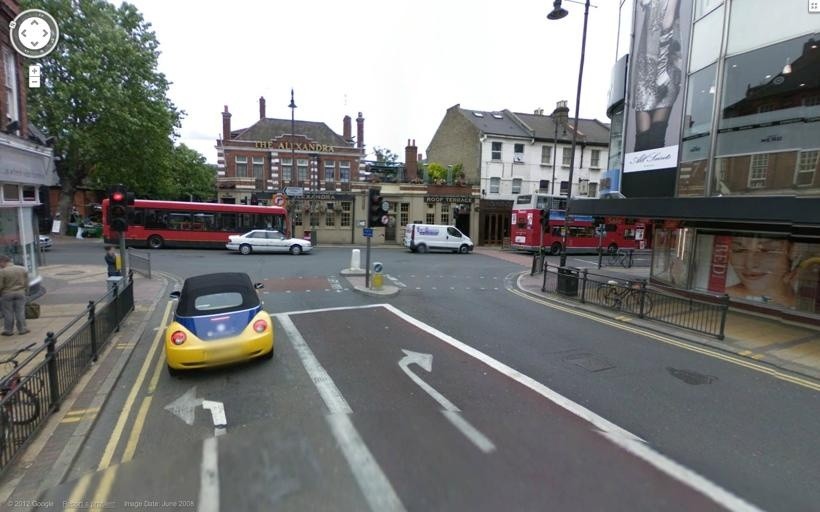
[164,273,275,374]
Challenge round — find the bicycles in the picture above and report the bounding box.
[0,343,41,451]
[597,278,653,316]
[608,250,635,268]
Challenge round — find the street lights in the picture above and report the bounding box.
[547,0,597,268]
[287,89,298,239]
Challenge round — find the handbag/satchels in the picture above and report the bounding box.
[25,303,40,319]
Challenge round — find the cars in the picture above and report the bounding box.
[34,233,53,250]
[224,229,313,255]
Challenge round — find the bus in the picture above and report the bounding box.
[101,197,289,252]
[510,193,648,256]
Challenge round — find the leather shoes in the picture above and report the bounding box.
[1,329,31,336]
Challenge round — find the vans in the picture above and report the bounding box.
[404,224,474,254]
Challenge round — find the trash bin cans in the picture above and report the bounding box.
[304,230,316,246]
[557,266,579,297]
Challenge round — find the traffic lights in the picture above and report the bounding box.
[107,183,129,232]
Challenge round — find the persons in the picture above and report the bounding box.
[632,0,682,152]
[104,244,122,276]
[725,237,799,308]
[0,252,30,336]
